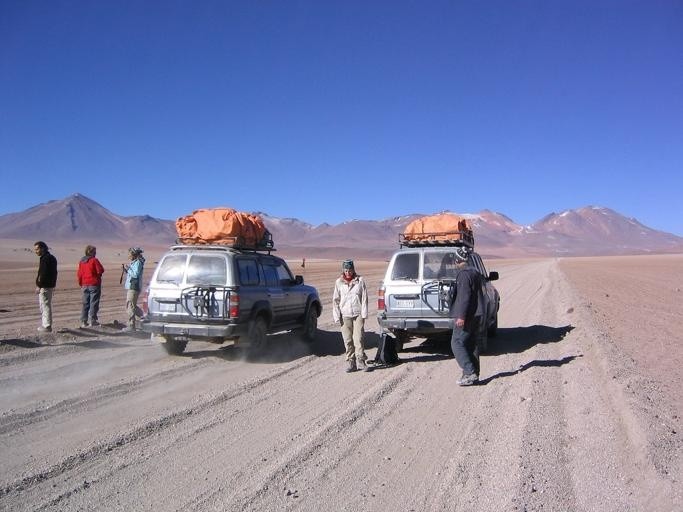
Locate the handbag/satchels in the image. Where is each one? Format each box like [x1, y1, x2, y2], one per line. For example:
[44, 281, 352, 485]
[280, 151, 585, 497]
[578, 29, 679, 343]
[374, 329, 400, 368]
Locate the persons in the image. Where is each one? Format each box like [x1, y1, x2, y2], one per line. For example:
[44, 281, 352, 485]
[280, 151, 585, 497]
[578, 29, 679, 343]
[76, 244, 103, 329]
[449, 246, 480, 386]
[331, 258, 370, 373]
[33, 241, 56, 333]
[119, 246, 145, 333]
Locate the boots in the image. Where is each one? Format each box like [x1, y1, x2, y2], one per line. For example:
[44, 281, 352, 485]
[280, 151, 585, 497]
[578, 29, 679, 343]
[356, 358, 370, 371]
[345, 358, 357, 372]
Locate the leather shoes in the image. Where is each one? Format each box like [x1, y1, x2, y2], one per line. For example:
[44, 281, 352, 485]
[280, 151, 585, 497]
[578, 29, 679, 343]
[89, 319, 99, 326]
[80, 320, 89, 328]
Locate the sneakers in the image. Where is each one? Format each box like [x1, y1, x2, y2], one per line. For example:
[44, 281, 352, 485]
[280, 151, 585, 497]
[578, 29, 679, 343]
[123, 327, 137, 333]
[455, 373, 480, 386]
[37, 325, 52, 332]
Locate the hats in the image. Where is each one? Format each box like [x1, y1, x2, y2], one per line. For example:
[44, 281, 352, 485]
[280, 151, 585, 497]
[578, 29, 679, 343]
[128, 246, 144, 255]
[342, 259, 354, 270]
[454, 245, 469, 262]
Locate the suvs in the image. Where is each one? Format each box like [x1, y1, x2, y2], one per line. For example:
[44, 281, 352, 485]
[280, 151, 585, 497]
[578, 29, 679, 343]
[137, 239, 325, 356]
[377, 233, 502, 354]
